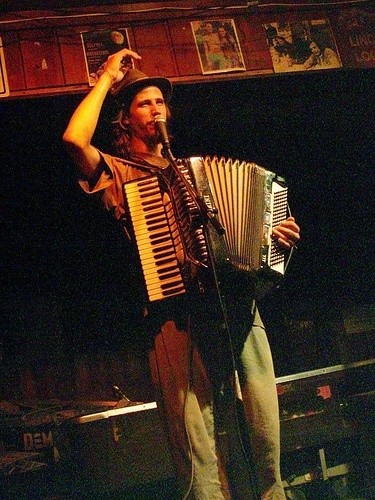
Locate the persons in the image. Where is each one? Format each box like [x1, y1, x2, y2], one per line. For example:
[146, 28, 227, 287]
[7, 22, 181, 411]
[202, 23, 243, 70]
[264, 20, 340, 69]
[61, 49, 301, 500]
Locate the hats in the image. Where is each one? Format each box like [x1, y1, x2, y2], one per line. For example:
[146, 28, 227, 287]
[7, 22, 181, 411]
[94, 68, 172, 137]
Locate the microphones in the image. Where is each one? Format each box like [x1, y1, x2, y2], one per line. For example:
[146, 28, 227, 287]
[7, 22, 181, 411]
[154, 114, 171, 149]
